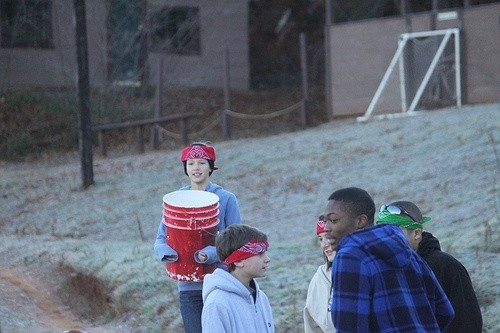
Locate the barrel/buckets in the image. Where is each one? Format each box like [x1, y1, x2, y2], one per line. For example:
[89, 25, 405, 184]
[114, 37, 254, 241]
[161, 190, 220, 283]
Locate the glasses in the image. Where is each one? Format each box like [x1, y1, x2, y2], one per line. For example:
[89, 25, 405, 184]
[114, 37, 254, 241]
[379, 204, 418, 223]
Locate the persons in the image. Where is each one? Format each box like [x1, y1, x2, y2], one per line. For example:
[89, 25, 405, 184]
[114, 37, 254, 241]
[154, 142, 241, 333]
[201, 224, 276, 333]
[323, 187, 455, 333]
[376, 201, 483, 333]
[304, 215, 336, 333]
